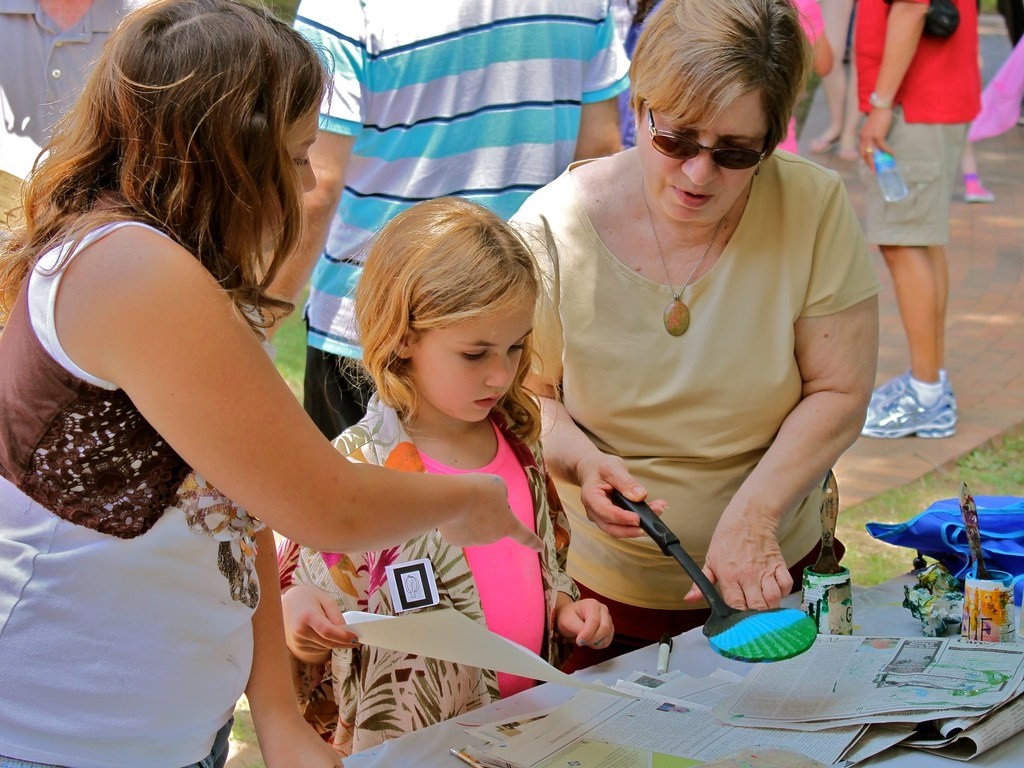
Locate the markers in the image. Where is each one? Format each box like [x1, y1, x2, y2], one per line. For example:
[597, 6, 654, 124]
[655, 632, 673, 675]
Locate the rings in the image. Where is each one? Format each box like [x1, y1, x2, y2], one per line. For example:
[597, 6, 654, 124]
[866, 148, 874, 153]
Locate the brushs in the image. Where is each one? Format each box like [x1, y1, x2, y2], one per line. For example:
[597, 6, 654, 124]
[812, 467, 844, 573]
[960, 482, 990, 578]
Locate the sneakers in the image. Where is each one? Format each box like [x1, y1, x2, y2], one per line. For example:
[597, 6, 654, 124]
[856, 366, 958, 438]
[964, 178, 996, 203]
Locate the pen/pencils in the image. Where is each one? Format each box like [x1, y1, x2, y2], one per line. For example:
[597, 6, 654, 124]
[449, 746, 486, 768]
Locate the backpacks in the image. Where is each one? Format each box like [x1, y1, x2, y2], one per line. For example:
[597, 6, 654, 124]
[865, 495, 1024, 608]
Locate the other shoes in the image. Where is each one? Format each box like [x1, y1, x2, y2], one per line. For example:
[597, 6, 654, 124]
[837, 142, 859, 160]
[809, 134, 843, 154]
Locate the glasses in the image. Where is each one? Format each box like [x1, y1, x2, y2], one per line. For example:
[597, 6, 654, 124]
[642, 99, 766, 170]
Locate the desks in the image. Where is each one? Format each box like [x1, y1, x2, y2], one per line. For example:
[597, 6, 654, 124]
[339, 563, 1023, 768]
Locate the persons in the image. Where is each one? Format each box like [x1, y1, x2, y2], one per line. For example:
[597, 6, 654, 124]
[0, 1, 546, 768]
[778, 0, 835, 154]
[812, 0, 864, 160]
[252, 3, 632, 442]
[852, 0, 983, 437]
[504, 1, 881, 675]
[1, 1, 127, 148]
[275, 196, 615, 761]
[960, 141, 997, 204]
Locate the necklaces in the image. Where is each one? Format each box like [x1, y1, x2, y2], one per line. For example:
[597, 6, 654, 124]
[642, 179, 723, 336]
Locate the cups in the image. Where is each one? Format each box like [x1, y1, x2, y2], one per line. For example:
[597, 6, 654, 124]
[1017, 589, 1024, 638]
[801, 565, 853, 636]
[962, 570, 1016, 643]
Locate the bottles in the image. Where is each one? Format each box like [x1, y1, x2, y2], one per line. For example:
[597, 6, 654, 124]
[871, 147, 908, 203]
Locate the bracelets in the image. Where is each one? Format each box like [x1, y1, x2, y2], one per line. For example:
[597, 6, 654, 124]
[869, 93, 893, 110]
